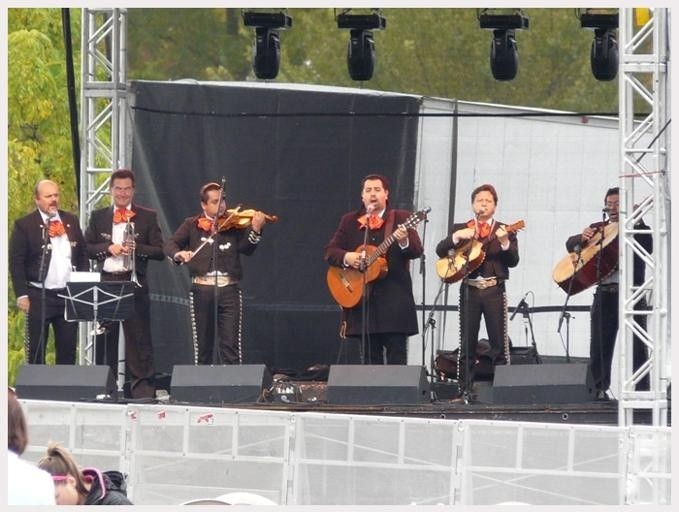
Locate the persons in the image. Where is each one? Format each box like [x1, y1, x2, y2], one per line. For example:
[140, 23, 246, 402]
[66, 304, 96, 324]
[324, 174, 424, 365]
[436, 184, 519, 365]
[566, 187, 653, 400]
[12, 180, 90, 365]
[8, 387, 134, 505]
[85, 169, 164, 397]
[162, 183, 267, 364]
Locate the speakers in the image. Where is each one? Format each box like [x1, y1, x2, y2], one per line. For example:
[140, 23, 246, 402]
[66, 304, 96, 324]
[492, 363, 597, 405]
[14, 364, 118, 404]
[171, 364, 273, 404]
[327, 364, 431, 405]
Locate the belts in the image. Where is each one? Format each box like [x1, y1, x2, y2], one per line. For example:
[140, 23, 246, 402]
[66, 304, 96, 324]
[463, 278, 497, 290]
[191, 274, 240, 287]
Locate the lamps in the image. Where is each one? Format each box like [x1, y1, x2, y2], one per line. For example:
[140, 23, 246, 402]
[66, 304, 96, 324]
[239, 8, 386, 82]
[475, 8, 620, 82]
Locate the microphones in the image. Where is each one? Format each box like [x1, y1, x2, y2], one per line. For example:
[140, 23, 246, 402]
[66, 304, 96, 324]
[423, 205, 432, 213]
[510, 293, 528, 321]
[602, 205, 610, 213]
[367, 204, 375, 213]
[475, 207, 485, 215]
[574, 245, 582, 253]
[46, 213, 56, 222]
[221, 176, 227, 198]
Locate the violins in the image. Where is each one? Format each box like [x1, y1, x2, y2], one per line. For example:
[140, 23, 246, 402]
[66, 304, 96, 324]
[216, 208, 278, 232]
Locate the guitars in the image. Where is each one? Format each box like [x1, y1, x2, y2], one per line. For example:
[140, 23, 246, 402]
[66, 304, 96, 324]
[436, 221, 524, 284]
[554, 205, 643, 295]
[327, 206, 431, 309]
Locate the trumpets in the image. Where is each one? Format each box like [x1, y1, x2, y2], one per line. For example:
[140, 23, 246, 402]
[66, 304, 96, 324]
[122, 215, 143, 289]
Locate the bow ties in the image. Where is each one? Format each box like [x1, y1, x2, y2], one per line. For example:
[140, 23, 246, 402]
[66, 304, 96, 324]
[112, 208, 136, 224]
[48, 220, 66, 238]
[197, 218, 212, 232]
[357, 213, 385, 230]
[466, 220, 491, 238]
